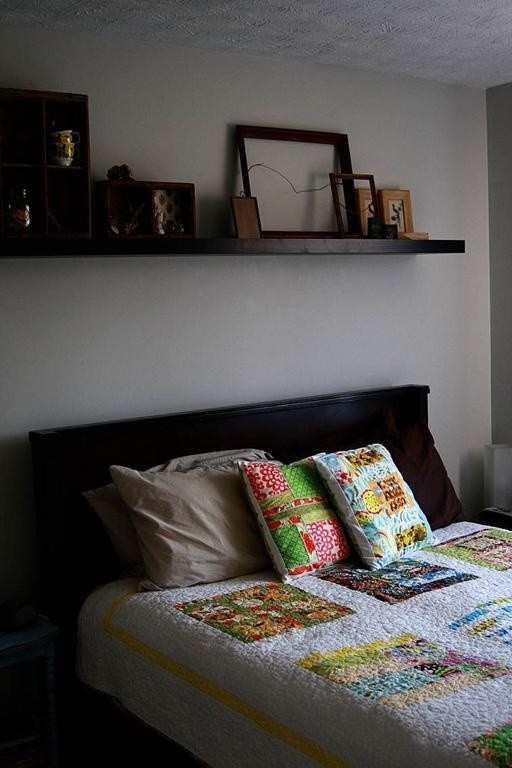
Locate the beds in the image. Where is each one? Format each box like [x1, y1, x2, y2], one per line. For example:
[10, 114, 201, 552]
[28, 383, 512, 768]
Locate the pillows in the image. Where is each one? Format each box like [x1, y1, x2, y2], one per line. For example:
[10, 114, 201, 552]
[237, 453, 354, 584]
[110, 459, 284, 592]
[83, 449, 272, 579]
[311, 444, 442, 572]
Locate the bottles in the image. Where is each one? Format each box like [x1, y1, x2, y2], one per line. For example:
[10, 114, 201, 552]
[9, 187, 32, 235]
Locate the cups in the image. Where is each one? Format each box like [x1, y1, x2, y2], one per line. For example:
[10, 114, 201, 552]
[49, 128, 81, 167]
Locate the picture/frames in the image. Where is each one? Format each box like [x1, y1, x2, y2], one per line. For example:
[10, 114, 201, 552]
[357, 188, 414, 240]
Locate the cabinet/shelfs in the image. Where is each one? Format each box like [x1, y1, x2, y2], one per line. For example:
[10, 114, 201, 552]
[0, 86, 94, 239]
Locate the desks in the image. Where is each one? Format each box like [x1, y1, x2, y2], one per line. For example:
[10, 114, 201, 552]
[0, 620, 62, 768]
